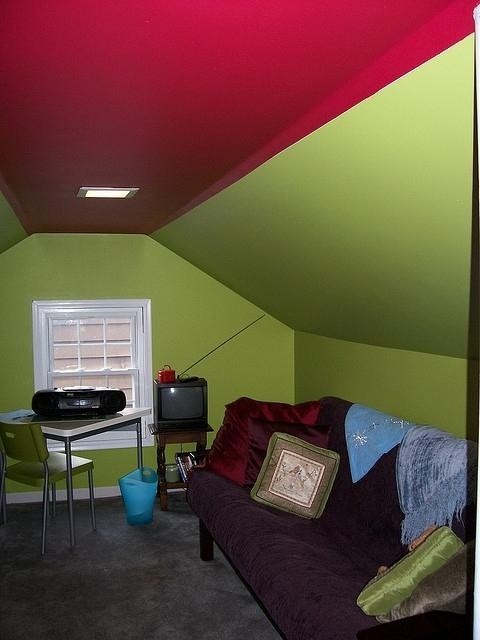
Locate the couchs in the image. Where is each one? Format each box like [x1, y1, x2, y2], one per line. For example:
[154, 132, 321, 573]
[187, 391, 473, 638]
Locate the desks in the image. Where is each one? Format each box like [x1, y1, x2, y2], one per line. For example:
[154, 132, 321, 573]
[148, 422, 213, 509]
[0, 408, 151, 546]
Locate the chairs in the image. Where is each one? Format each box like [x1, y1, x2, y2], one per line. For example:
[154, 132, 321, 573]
[0, 420, 98, 555]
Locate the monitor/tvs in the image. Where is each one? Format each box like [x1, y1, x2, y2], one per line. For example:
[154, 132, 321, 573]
[153, 380, 208, 430]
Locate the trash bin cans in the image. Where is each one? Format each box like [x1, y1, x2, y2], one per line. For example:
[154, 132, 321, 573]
[119, 468, 158, 526]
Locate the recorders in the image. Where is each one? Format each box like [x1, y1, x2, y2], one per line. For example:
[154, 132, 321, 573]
[32, 385, 126, 416]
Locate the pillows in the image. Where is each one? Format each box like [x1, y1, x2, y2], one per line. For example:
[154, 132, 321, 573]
[353, 525, 467, 624]
[248, 431, 343, 521]
